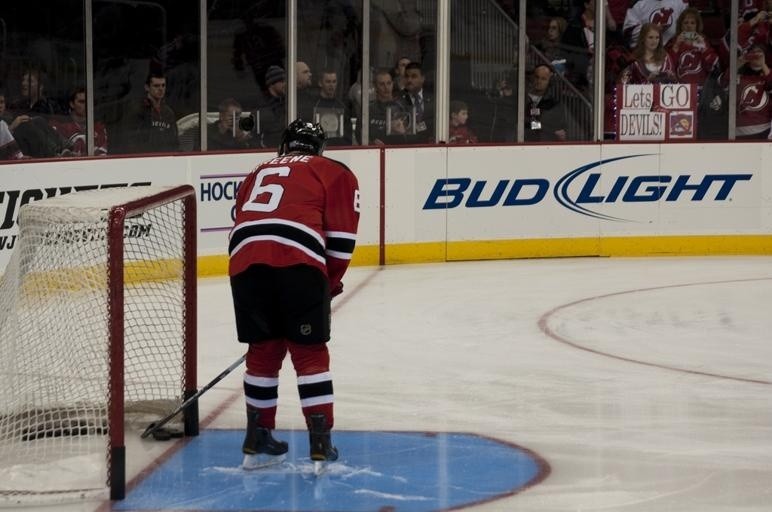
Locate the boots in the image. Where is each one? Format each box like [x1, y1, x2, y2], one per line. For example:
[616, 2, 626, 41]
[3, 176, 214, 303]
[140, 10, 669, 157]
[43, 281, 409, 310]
[309, 414, 339, 462]
[242, 411, 289, 455]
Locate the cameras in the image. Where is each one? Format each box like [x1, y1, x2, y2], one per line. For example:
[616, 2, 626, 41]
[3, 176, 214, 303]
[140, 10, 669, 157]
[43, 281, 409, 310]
[681, 32, 696, 41]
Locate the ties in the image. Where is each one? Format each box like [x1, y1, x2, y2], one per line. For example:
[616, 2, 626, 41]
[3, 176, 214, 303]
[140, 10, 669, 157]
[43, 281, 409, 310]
[412, 94, 424, 122]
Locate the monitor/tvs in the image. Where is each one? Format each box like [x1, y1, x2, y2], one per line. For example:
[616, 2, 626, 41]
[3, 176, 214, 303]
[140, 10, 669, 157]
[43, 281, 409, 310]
[234, 111, 257, 135]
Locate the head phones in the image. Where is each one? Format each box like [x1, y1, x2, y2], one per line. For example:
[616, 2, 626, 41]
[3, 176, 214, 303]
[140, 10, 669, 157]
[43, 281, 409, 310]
[529, 63, 556, 88]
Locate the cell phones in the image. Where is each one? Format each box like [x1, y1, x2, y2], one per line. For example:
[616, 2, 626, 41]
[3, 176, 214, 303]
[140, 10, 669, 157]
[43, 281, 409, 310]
[745, 52, 760, 60]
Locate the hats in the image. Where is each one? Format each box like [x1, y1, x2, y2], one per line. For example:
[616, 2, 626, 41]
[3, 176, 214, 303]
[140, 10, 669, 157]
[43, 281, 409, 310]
[264, 65, 286, 85]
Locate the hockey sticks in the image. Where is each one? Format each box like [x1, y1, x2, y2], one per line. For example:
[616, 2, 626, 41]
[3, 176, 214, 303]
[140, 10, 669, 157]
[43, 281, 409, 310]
[142, 353, 247, 438]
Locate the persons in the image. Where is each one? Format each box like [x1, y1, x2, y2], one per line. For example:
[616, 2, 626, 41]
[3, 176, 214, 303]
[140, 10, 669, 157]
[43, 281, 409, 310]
[227, 118, 359, 461]
[1, 1, 770, 158]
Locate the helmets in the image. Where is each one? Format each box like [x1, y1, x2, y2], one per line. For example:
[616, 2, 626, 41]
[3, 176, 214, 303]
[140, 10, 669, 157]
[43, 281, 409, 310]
[278, 118, 328, 158]
